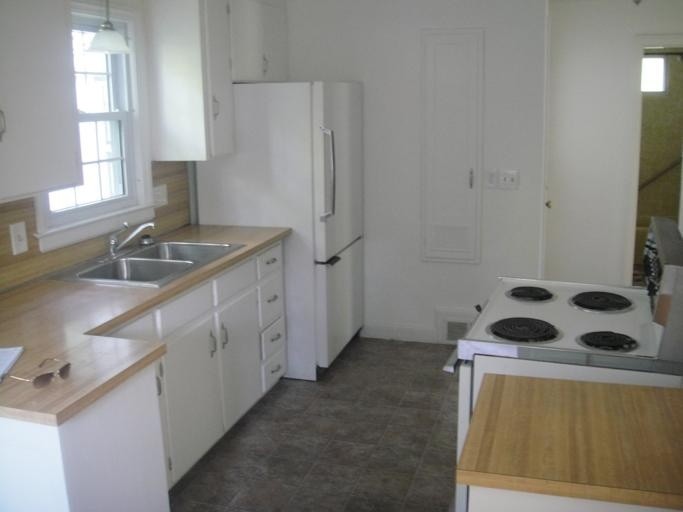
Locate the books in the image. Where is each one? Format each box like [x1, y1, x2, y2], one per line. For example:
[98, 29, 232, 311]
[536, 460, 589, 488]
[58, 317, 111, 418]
[0, 346, 25, 380]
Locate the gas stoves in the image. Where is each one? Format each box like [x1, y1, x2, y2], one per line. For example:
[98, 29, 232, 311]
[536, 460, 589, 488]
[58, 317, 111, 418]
[466, 275, 657, 359]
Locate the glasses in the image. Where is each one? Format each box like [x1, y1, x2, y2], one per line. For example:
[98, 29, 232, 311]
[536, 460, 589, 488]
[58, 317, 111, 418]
[10, 357, 71, 388]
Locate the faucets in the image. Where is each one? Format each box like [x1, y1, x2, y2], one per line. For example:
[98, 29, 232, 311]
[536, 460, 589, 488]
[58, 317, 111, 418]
[107, 221, 159, 258]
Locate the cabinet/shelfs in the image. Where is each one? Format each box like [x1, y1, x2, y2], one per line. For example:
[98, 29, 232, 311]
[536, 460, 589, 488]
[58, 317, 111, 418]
[229, 0, 289, 83]
[143, 0, 235, 163]
[0, 0, 83, 204]
[420, 25, 486, 266]
[0, 239, 289, 511]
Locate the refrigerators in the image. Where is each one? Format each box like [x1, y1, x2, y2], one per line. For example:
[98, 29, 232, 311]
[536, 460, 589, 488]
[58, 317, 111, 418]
[196, 83, 367, 382]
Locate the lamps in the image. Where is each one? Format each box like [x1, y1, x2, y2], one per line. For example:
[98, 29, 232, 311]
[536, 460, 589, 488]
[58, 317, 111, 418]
[86, 0, 132, 54]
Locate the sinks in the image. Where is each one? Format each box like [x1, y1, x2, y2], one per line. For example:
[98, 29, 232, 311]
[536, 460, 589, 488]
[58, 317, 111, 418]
[121, 240, 233, 264]
[77, 257, 189, 286]
